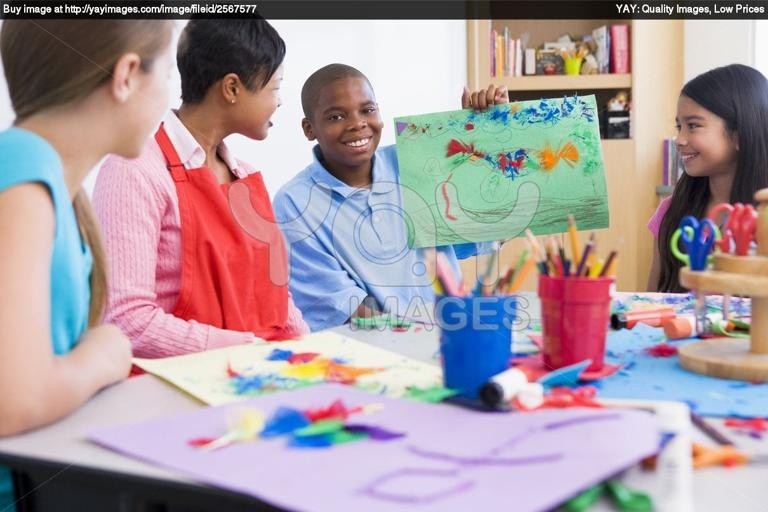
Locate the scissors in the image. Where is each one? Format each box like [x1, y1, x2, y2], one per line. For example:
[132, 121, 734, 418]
[669, 200, 758, 336]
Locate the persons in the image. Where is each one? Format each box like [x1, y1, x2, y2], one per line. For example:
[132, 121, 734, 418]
[273, 58, 512, 334]
[645, 63, 767, 294]
[0, 14, 184, 511]
[91, 17, 312, 381]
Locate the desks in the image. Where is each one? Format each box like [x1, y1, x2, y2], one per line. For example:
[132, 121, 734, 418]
[1, 294, 767, 512]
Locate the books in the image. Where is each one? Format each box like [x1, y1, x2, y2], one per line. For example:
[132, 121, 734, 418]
[489, 22, 630, 77]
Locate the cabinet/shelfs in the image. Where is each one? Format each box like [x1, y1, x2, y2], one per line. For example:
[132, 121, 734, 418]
[467, 20, 685, 292]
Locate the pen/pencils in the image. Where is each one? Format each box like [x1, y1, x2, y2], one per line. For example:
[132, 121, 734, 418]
[426, 213, 619, 296]
[691, 410, 732, 445]
